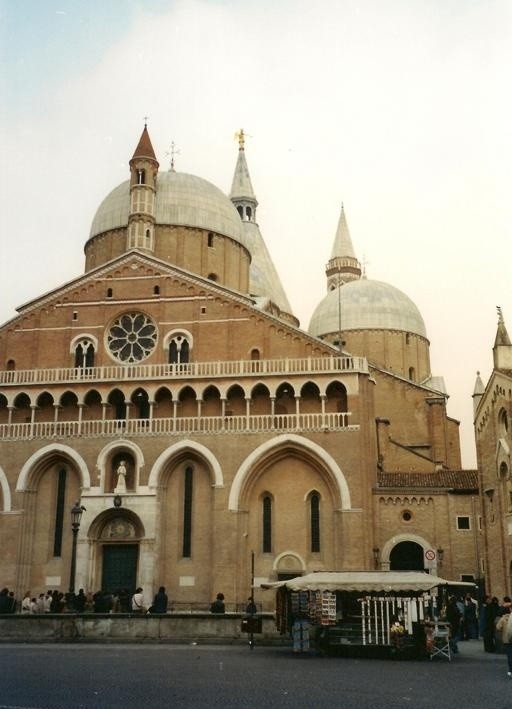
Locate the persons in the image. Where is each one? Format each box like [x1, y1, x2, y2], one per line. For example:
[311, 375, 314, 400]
[441, 591, 511, 677]
[114, 460, 128, 493]
[1, 586, 132, 615]
[131, 587, 146, 613]
[246, 596, 257, 613]
[147, 586, 169, 614]
[208, 592, 224, 615]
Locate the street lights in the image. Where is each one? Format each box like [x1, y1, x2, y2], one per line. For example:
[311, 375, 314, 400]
[67, 500, 87, 613]
